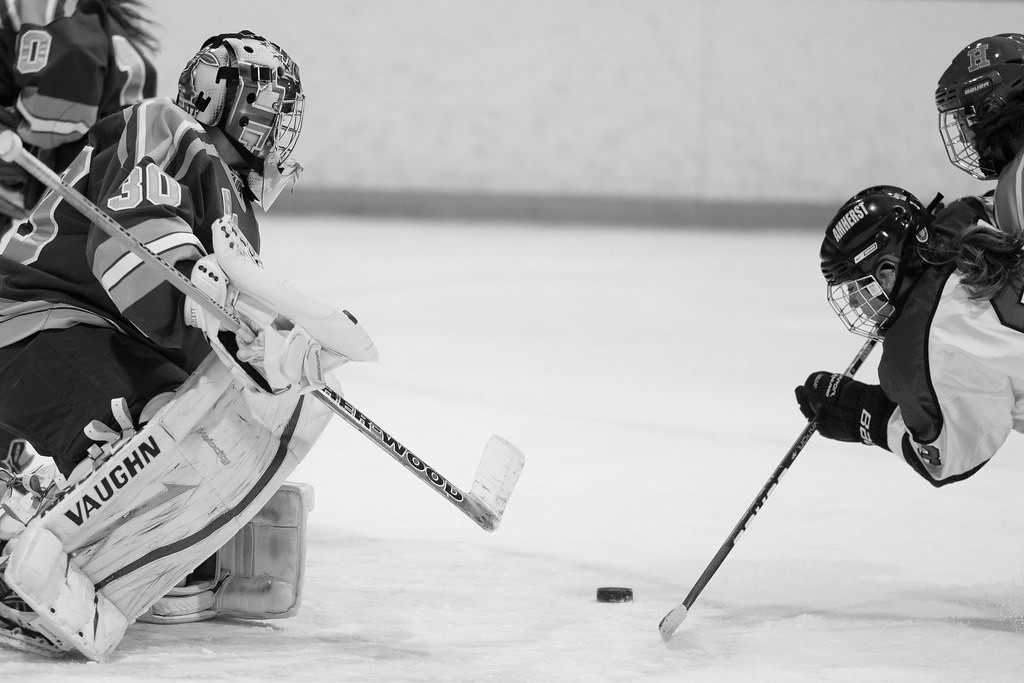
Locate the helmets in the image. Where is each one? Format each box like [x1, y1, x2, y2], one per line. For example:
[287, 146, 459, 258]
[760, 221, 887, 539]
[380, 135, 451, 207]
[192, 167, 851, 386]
[935, 33, 1024, 180]
[172, 30, 305, 213]
[819, 186, 936, 342]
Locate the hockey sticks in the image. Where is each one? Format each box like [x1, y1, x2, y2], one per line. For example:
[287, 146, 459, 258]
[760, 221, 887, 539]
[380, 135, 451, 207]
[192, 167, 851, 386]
[0, 124, 529, 536]
[657, 320, 890, 646]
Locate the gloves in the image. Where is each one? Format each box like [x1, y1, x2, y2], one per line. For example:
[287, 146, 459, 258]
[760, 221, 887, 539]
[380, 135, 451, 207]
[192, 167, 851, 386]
[795, 371, 898, 453]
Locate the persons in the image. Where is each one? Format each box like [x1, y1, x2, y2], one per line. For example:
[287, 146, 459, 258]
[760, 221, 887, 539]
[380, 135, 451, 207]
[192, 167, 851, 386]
[796, 31, 1024, 487]
[1, 0, 381, 661]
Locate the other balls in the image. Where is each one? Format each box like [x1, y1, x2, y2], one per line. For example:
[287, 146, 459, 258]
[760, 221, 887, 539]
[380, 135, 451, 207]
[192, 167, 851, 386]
[597, 587, 634, 604]
